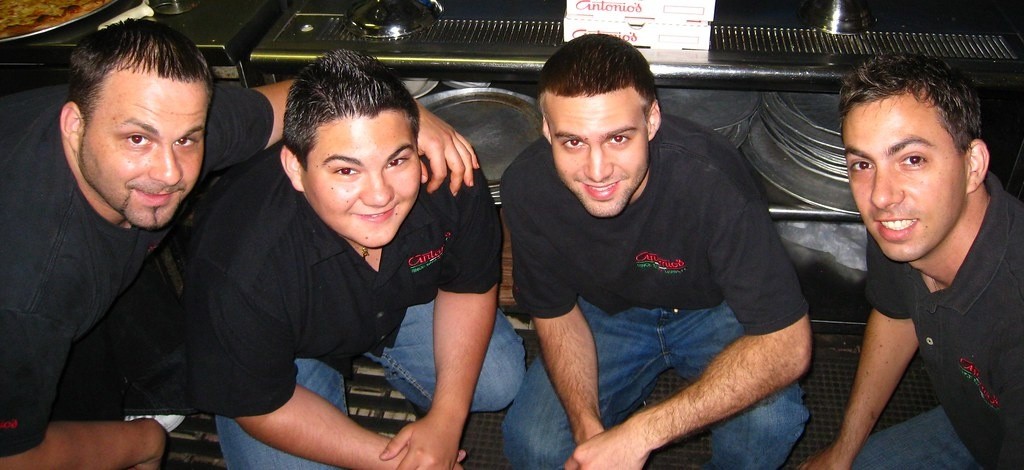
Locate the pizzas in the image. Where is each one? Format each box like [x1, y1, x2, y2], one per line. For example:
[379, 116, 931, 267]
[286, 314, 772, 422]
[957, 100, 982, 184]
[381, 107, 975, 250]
[0, 0, 110, 37]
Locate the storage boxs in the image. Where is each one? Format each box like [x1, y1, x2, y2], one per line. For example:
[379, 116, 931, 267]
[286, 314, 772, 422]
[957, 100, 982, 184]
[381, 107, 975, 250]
[564, 0, 716, 50]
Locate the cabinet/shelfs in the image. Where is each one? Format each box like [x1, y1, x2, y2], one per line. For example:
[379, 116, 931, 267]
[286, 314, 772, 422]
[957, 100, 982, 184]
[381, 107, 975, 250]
[250, 0, 1024, 335]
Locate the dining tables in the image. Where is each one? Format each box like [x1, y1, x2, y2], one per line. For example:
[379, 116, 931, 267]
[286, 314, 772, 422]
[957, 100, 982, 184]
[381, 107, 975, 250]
[0, 0, 282, 97]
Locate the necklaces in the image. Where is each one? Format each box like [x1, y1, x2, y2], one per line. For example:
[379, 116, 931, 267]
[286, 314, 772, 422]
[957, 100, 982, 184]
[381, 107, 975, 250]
[362, 246, 370, 260]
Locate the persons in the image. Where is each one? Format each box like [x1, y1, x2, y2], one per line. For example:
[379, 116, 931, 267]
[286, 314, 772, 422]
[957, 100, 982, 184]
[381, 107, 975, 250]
[184, 49, 526, 470]
[499, 33, 812, 470]
[798, 53, 1024, 470]
[0, 19, 479, 470]
